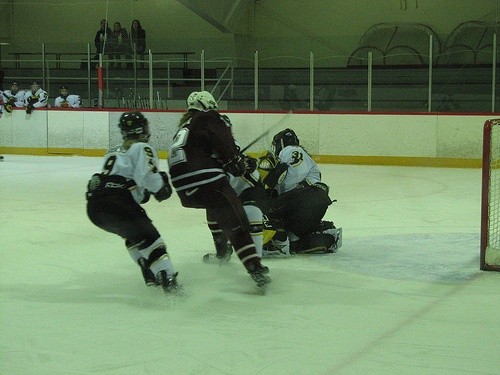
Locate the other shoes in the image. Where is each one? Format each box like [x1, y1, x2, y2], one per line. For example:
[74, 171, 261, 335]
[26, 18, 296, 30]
[137, 258, 158, 287]
[156, 269, 184, 297]
[263, 237, 290, 259]
[322, 220, 343, 254]
[203, 250, 234, 266]
[248, 263, 272, 289]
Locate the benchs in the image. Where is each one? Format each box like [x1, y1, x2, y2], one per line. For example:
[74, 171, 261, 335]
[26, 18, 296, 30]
[8, 52, 197, 70]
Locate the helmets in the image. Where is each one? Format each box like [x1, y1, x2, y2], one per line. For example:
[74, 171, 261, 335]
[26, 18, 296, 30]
[117, 111, 150, 139]
[271, 128, 300, 157]
[187, 91, 218, 112]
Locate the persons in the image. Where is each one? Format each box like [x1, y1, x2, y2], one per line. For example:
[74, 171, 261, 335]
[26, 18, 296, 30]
[228, 128, 343, 257]
[85, 112, 192, 311]
[55, 85, 81, 108]
[23, 81, 51, 114]
[89, 19, 146, 73]
[168, 91, 272, 295]
[1, 81, 26, 113]
